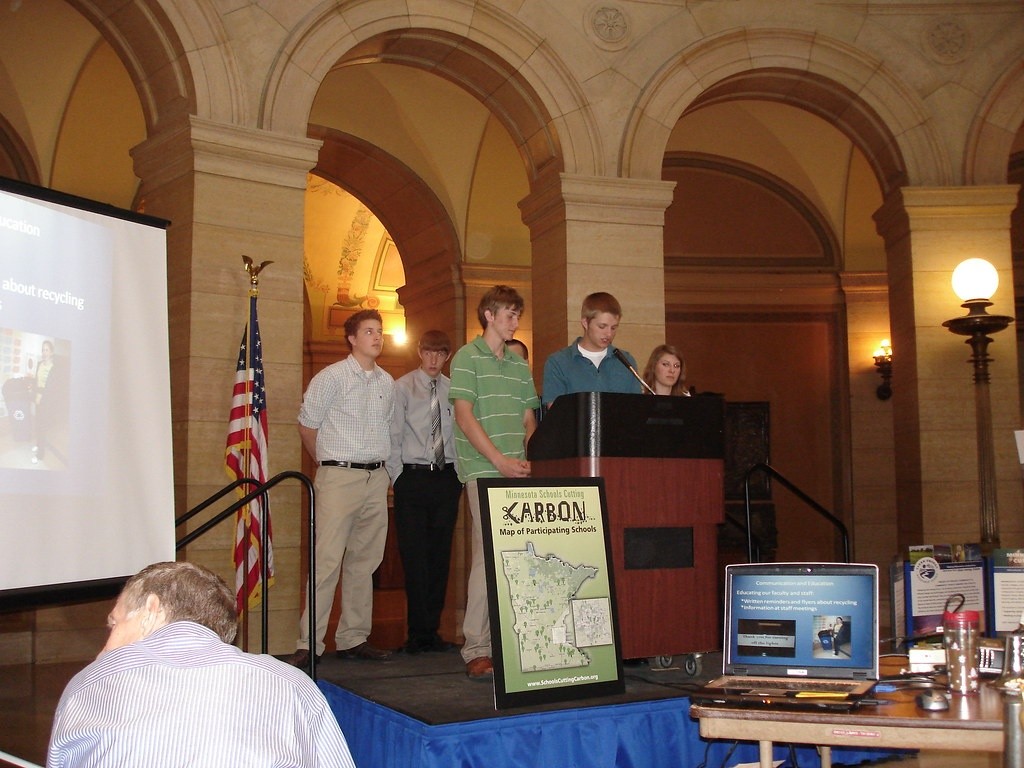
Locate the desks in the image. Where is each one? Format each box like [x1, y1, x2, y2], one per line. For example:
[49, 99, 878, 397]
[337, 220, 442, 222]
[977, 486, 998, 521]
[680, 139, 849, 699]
[691, 652, 1023, 768]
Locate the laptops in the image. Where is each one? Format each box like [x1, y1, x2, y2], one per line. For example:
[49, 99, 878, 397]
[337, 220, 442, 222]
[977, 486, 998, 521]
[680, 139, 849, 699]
[689, 562, 879, 713]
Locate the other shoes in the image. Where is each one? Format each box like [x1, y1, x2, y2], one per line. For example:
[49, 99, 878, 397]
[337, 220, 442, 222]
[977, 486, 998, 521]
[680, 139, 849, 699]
[397, 640, 426, 656]
[426, 635, 456, 653]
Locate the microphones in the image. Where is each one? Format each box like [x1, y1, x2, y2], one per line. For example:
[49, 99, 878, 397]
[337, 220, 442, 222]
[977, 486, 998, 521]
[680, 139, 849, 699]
[613, 348, 656, 395]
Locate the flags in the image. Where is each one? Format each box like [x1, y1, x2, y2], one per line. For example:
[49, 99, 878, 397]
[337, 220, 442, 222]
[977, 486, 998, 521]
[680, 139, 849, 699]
[225, 288, 275, 626]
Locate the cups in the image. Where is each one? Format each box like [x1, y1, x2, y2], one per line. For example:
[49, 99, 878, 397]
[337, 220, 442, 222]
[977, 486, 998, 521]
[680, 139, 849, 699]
[943, 611, 979, 693]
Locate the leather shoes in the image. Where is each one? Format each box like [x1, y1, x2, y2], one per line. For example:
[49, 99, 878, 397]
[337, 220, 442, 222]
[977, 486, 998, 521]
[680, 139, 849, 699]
[286, 649, 321, 669]
[336, 643, 393, 660]
[466, 657, 494, 681]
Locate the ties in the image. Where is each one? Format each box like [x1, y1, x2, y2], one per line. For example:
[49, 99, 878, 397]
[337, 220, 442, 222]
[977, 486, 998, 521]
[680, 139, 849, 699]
[429, 379, 445, 470]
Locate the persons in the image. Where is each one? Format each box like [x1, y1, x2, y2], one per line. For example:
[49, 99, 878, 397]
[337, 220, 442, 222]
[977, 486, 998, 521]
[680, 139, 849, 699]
[384, 330, 460, 653]
[46, 562, 356, 768]
[28, 341, 62, 464]
[831, 617, 846, 656]
[641, 344, 690, 396]
[505, 339, 528, 365]
[541, 293, 641, 409]
[287, 309, 396, 668]
[447, 283, 541, 679]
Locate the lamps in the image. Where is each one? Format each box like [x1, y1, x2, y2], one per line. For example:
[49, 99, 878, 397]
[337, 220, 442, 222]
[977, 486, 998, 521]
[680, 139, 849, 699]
[872, 338, 893, 399]
[942, 259, 1015, 540]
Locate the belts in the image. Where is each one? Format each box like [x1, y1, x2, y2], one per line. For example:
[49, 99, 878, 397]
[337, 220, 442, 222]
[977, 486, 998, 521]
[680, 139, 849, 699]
[322, 460, 385, 470]
[405, 463, 454, 471]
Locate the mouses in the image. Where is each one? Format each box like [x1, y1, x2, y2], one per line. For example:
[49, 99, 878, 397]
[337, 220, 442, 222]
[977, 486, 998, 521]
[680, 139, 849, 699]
[915, 689, 949, 711]
